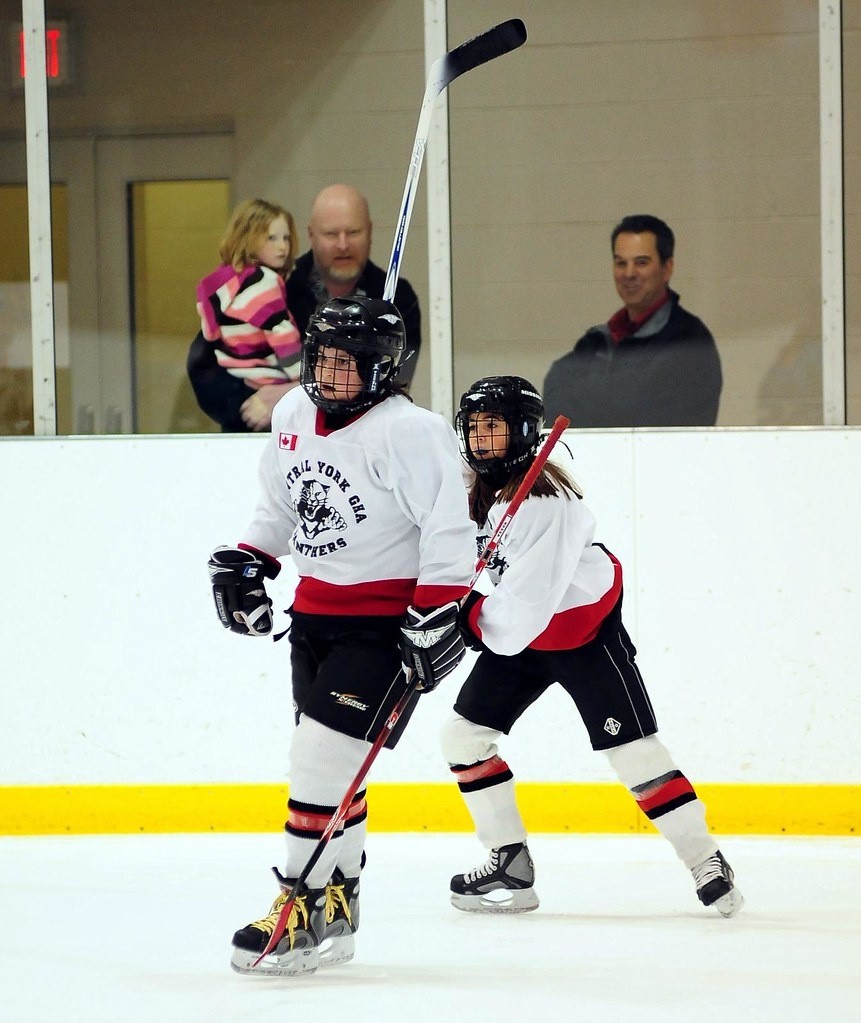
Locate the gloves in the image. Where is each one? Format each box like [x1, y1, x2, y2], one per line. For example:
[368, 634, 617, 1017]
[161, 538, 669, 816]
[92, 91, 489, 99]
[396, 601, 467, 693]
[457, 587, 489, 652]
[207, 544, 282, 636]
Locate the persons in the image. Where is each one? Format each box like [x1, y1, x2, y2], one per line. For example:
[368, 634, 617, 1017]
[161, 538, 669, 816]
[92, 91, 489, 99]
[441, 377, 745, 919]
[196, 199, 306, 389]
[542, 214, 722, 429]
[186, 183, 422, 435]
[206, 296, 475, 977]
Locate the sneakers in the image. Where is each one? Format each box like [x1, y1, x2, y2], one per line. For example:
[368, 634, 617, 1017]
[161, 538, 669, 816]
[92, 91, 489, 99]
[690, 849, 744, 918]
[230, 867, 326, 977]
[317, 850, 366, 969]
[450, 840, 539, 912]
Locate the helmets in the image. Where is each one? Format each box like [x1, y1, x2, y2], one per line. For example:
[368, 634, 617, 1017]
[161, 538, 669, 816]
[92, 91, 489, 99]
[455, 375, 545, 475]
[299, 295, 407, 415]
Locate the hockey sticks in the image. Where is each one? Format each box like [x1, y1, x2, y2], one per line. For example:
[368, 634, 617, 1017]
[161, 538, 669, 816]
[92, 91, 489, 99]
[382, 17, 528, 301]
[251, 414, 570, 969]
